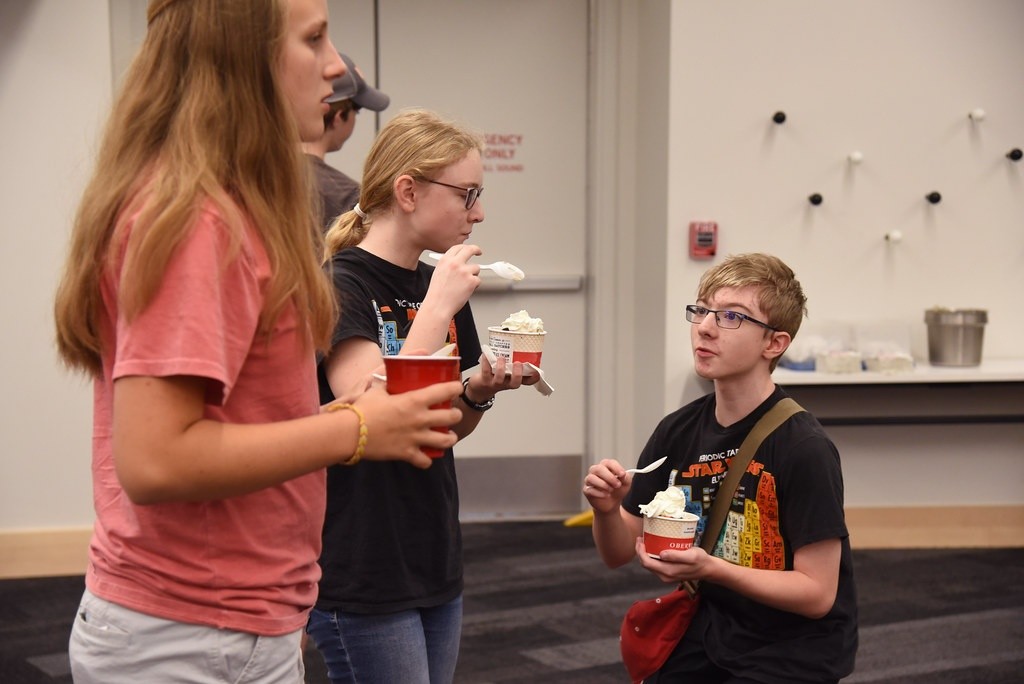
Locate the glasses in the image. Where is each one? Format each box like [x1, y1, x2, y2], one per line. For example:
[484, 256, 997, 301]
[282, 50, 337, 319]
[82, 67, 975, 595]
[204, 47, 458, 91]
[413, 175, 484, 211]
[685, 304, 782, 335]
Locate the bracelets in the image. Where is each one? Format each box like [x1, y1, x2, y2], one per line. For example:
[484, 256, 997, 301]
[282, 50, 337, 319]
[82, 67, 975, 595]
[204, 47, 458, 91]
[461, 377, 495, 412]
[328, 404, 367, 465]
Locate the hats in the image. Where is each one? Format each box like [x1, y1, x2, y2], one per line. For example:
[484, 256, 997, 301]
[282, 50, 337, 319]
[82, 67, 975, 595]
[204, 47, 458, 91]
[320, 52, 389, 111]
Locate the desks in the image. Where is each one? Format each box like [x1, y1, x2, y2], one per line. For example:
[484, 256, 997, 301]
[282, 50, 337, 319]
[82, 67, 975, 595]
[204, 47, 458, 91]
[769, 359, 1024, 425]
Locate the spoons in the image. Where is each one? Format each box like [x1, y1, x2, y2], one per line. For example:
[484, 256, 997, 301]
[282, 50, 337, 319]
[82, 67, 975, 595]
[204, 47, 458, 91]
[586, 456, 667, 490]
[429, 253, 524, 281]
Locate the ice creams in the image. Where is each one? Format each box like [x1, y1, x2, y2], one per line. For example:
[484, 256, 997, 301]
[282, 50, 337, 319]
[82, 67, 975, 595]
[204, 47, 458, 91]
[637, 486, 686, 519]
[501, 309, 544, 334]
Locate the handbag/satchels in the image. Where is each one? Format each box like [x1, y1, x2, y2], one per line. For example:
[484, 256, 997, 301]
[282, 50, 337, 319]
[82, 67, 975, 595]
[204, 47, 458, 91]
[619, 580, 699, 683]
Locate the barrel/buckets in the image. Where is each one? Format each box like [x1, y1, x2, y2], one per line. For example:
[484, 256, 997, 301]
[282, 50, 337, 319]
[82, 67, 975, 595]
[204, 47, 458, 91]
[924, 309, 987, 367]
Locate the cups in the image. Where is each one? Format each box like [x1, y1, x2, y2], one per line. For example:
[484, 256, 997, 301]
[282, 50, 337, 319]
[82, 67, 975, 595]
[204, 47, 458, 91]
[643, 512, 700, 559]
[383, 356, 461, 457]
[488, 327, 547, 376]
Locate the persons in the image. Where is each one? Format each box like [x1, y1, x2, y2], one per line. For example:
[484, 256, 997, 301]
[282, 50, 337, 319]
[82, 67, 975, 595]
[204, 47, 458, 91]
[583, 253, 859, 684]
[307, 110, 542, 684]
[50, 0, 466, 684]
[301, 53, 390, 250]
